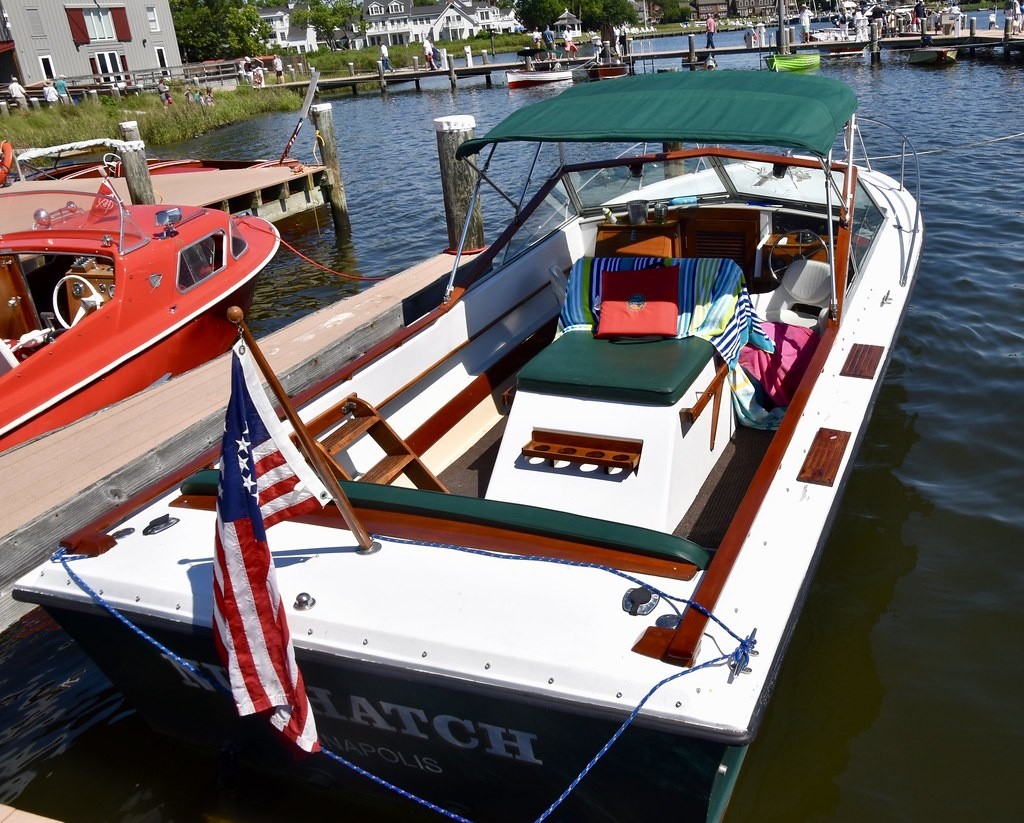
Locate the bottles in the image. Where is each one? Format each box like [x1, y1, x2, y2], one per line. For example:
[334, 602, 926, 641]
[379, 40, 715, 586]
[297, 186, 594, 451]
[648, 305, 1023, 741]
[603, 207, 616, 223]
[669, 197, 697, 205]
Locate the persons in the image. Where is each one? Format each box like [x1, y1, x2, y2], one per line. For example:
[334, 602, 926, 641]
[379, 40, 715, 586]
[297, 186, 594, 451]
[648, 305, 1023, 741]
[8, 77, 31, 112]
[600, 21, 628, 57]
[704, 13, 716, 49]
[272, 53, 286, 84]
[380, 42, 396, 74]
[244, 56, 254, 87]
[183, 87, 217, 107]
[798, 4, 814, 43]
[158, 78, 173, 114]
[1012, 0, 1024, 35]
[43, 75, 75, 106]
[422, 38, 440, 70]
[833, 0, 931, 43]
[532, 24, 578, 62]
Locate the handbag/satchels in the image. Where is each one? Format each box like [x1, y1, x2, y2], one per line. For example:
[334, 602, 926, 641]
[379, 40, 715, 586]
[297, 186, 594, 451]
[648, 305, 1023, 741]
[1013, 19, 1018, 27]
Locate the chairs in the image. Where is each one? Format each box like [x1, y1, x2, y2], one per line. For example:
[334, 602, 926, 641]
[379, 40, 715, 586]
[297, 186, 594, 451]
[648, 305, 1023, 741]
[750, 259, 831, 334]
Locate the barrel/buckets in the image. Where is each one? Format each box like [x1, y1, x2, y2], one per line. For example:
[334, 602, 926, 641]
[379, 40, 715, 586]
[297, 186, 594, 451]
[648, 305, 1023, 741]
[626, 200, 649, 224]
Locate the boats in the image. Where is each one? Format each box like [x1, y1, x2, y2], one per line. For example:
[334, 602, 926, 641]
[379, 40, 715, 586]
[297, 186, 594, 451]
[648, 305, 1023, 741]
[0, 139, 284, 456]
[9, 68, 925, 823]
[906, 33, 958, 65]
[505, 48, 573, 88]
[763, 52, 821, 73]
[584, 60, 630, 81]
[801, 5, 964, 55]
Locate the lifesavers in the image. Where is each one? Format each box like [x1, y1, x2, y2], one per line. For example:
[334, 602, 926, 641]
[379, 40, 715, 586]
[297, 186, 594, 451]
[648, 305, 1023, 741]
[0, 140, 13, 186]
[744, 32, 758, 46]
[253, 72, 263, 85]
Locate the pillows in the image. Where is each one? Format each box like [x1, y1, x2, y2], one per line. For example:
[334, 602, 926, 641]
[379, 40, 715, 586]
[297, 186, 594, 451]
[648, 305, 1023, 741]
[597, 261, 682, 337]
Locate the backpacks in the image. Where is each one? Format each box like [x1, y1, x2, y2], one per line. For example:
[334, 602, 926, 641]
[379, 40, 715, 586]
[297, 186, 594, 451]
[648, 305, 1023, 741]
[840, 13, 846, 24]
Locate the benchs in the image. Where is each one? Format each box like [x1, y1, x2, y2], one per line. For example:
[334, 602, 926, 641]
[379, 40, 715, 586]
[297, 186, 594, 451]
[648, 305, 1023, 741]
[539, 244, 747, 360]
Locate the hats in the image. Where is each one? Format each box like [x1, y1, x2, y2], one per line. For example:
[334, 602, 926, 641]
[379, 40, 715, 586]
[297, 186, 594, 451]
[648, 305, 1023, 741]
[800, 4, 807, 9]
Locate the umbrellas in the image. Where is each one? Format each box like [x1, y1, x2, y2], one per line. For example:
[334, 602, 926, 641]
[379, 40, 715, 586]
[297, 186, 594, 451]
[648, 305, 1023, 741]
[838, 1, 857, 16]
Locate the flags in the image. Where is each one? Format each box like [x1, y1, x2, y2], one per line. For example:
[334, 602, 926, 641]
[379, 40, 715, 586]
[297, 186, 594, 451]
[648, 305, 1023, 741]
[212, 341, 334, 754]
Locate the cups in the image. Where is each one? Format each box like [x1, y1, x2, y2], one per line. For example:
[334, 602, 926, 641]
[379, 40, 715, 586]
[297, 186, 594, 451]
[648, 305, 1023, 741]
[655, 204, 668, 224]
